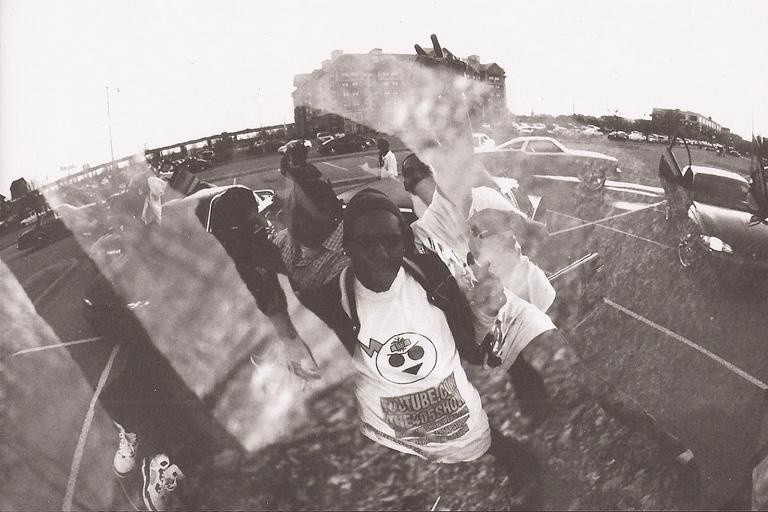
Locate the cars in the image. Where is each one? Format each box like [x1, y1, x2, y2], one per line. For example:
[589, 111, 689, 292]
[506, 119, 602, 145]
[352, 134, 375, 148]
[1, 146, 218, 251]
[316, 137, 371, 154]
[655, 135, 767, 288]
[83, 182, 283, 333]
[81, 179, 215, 269]
[472, 131, 495, 155]
[606, 128, 743, 158]
[474, 136, 623, 182]
[233, 136, 286, 156]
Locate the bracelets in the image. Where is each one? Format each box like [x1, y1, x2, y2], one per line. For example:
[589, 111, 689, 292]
[670, 447, 691, 473]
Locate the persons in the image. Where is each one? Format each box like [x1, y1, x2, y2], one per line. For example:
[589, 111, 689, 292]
[130, 32, 700, 511]
[110, 418, 182, 511]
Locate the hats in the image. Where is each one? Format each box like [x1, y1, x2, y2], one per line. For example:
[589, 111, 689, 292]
[342, 187, 409, 239]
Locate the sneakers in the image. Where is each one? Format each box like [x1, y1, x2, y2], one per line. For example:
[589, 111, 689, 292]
[112, 430, 141, 479]
[141, 450, 186, 512]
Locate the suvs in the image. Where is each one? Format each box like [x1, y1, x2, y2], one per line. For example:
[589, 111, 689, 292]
[277, 139, 312, 156]
[316, 131, 346, 142]
